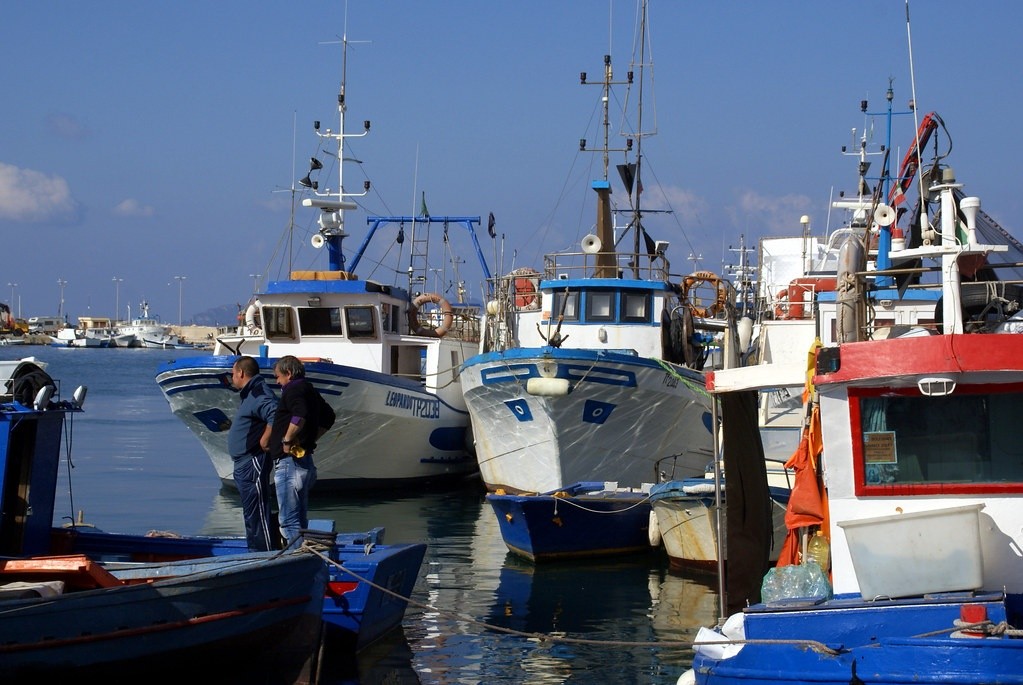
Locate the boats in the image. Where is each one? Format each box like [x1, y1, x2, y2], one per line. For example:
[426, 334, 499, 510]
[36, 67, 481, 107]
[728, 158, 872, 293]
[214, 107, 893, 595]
[680, 169, 1023, 685]
[155, 0, 488, 500]
[704, 92, 1023, 507]
[0, 525, 330, 685]
[458, 0, 722, 561]
[0, 353, 427, 639]
[39, 320, 179, 348]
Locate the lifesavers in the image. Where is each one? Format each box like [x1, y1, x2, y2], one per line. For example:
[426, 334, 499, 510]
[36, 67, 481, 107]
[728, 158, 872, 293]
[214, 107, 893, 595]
[775, 288, 790, 320]
[680, 270, 728, 318]
[407, 292, 454, 339]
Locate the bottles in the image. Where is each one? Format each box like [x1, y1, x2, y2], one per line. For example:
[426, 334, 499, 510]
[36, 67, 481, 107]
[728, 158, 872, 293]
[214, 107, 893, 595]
[807, 531, 829, 573]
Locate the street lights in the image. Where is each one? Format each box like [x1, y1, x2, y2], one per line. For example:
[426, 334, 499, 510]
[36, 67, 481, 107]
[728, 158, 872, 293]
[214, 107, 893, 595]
[5, 281, 17, 317]
[687, 256, 705, 273]
[57, 279, 68, 326]
[175, 275, 186, 325]
[112, 276, 124, 327]
[430, 266, 443, 309]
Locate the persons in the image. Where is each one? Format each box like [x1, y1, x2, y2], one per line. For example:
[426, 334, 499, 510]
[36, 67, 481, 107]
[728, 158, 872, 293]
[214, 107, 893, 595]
[228, 356, 280, 554]
[267, 356, 336, 552]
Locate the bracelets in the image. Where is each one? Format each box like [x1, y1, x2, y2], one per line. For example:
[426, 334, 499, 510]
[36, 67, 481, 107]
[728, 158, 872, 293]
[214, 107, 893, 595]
[282, 438, 292, 445]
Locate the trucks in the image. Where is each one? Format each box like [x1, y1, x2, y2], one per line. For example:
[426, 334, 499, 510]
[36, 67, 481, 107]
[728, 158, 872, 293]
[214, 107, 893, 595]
[0, 318, 28, 336]
[28, 316, 58, 335]
